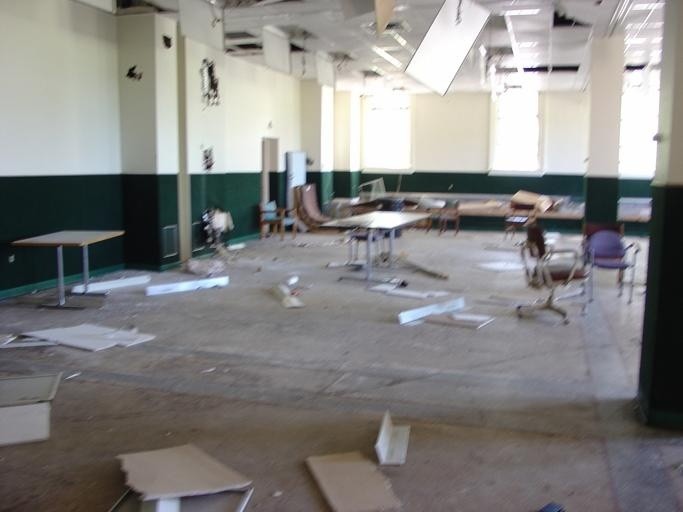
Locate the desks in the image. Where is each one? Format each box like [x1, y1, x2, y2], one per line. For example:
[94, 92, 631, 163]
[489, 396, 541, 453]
[11, 228, 126, 311]
[319, 210, 432, 286]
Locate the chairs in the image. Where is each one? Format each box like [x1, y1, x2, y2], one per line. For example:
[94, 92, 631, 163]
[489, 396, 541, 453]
[259, 188, 541, 240]
[347, 207, 384, 265]
[516, 216, 588, 326]
[582, 220, 641, 303]
